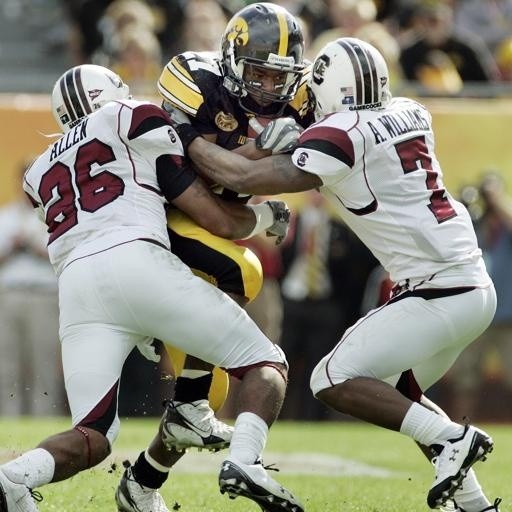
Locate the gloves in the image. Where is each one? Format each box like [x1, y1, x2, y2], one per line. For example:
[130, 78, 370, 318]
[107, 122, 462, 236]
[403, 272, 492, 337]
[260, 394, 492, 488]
[265, 198, 290, 246]
[161, 101, 205, 157]
[135, 336, 164, 363]
[255, 115, 301, 156]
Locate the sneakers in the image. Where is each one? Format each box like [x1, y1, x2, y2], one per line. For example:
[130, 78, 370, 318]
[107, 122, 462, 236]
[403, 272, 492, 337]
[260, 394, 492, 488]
[451, 497, 503, 512]
[114, 467, 172, 512]
[0, 467, 40, 512]
[159, 398, 233, 453]
[427, 416, 494, 509]
[218, 458, 303, 512]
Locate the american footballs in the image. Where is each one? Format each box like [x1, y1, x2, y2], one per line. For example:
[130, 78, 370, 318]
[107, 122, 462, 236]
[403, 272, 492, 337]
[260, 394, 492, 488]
[247, 116, 304, 145]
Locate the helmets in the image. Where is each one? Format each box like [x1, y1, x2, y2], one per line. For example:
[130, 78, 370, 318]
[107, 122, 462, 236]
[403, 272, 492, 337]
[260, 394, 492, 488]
[218, 1, 303, 120]
[307, 37, 391, 120]
[48, 64, 130, 135]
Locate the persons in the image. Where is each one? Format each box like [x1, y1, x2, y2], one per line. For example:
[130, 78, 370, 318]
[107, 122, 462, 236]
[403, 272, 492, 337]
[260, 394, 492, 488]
[163, 38, 503, 512]
[1, 1, 511, 99]
[114, 1, 309, 512]
[0, 62, 307, 512]
